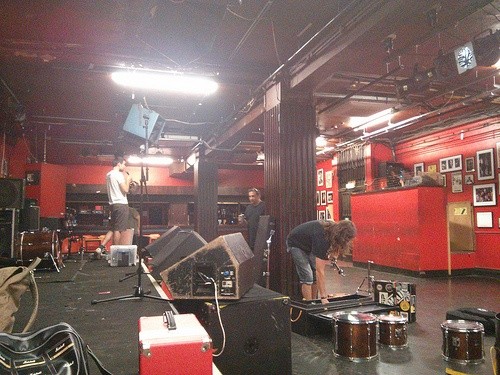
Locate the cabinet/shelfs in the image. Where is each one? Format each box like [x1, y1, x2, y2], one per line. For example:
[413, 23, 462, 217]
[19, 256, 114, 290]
[64, 195, 250, 234]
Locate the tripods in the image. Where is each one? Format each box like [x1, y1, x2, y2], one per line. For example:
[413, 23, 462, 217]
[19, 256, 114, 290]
[90, 151, 173, 306]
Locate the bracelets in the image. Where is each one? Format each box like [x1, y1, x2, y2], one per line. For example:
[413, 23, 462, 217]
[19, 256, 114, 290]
[320, 296, 327, 299]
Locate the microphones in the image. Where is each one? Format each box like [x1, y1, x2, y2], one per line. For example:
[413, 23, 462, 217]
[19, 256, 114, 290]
[126, 170, 130, 175]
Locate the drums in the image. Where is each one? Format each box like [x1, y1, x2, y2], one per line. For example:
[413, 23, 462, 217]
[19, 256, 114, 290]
[19, 230, 62, 270]
[377, 313, 409, 349]
[439, 319, 485, 366]
[332, 313, 378, 363]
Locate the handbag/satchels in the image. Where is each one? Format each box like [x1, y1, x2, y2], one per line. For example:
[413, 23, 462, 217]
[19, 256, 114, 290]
[0, 254, 112, 375]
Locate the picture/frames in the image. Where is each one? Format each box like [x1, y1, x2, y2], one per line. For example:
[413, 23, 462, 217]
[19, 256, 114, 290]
[476, 148, 495, 180]
[413, 162, 425, 177]
[316, 191, 320, 206]
[327, 191, 333, 203]
[326, 207, 333, 221]
[321, 190, 327, 205]
[317, 168, 325, 186]
[438, 154, 463, 174]
[465, 156, 475, 172]
[472, 183, 497, 206]
[318, 210, 326, 221]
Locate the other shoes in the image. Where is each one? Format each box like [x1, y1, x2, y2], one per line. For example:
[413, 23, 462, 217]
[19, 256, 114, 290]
[96, 245, 108, 257]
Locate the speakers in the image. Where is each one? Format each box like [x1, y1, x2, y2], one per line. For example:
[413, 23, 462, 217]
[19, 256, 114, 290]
[0, 177, 40, 231]
[144, 226, 256, 300]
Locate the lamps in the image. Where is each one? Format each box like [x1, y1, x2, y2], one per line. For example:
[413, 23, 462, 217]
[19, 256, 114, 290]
[394, 30, 500, 102]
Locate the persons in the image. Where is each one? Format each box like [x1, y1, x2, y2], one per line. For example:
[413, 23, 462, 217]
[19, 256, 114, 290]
[106, 156, 132, 245]
[286, 219, 356, 304]
[239, 187, 266, 252]
[93, 207, 141, 260]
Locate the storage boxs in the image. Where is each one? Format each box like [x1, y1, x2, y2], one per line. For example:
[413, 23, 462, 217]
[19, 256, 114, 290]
[110, 244, 137, 267]
